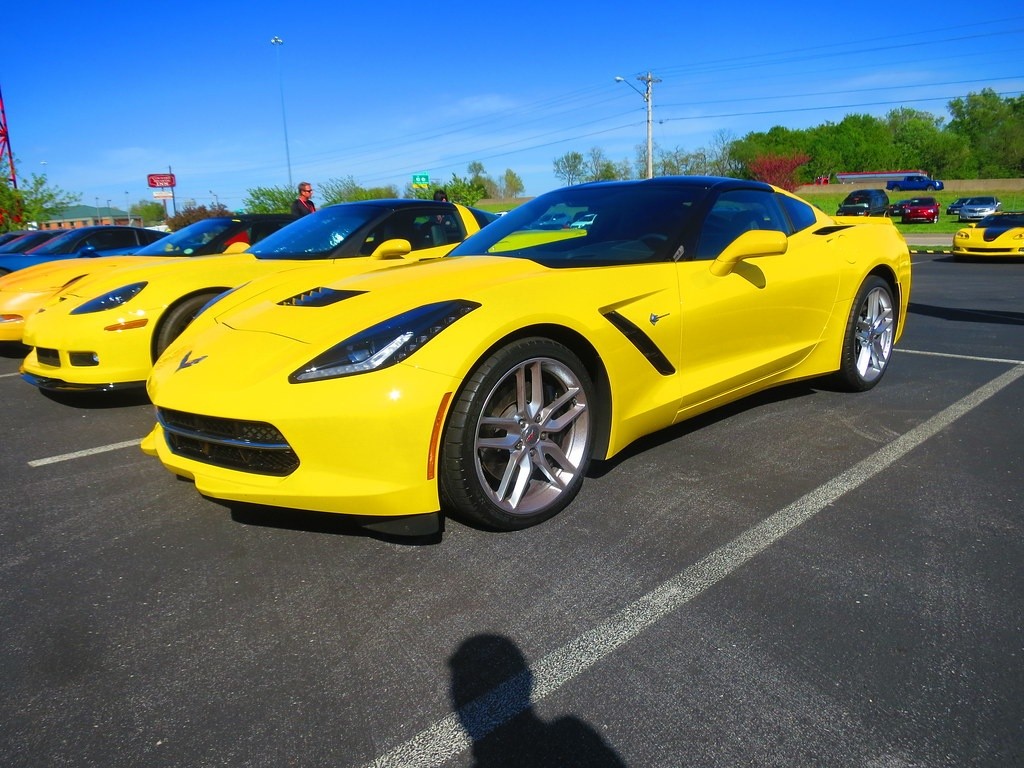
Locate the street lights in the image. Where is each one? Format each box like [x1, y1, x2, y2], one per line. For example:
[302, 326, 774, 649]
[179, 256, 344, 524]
[615, 76, 653, 178]
[271, 36, 294, 191]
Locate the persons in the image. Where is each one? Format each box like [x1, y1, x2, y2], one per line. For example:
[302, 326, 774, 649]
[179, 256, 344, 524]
[291, 182, 316, 217]
[429, 189, 452, 226]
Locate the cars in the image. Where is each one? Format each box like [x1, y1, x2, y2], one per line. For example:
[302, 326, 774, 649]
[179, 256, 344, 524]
[959, 197, 1001, 221]
[889, 200, 910, 216]
[531, 212, 571, 229]
[570, 213, 596, 229]
[947, 197, 972, 214]
[902, 197, 940, 223]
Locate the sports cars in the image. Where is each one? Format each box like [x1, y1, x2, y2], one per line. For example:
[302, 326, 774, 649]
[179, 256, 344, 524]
[0, 200, 588, 394]
[951, 211, 1024, 258]
[139, 174, 912, 534]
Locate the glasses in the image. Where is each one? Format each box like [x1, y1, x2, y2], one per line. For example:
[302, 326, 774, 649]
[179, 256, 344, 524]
[302, 189, 312, 193]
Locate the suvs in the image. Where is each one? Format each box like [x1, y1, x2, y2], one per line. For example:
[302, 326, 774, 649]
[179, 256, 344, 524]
[836, 189, 889, 216]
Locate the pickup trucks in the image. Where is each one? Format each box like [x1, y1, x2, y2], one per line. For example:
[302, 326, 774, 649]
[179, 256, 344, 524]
[885, 175, 944, 192]
